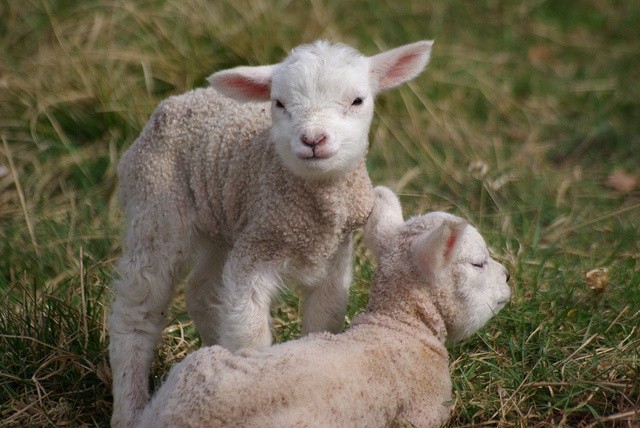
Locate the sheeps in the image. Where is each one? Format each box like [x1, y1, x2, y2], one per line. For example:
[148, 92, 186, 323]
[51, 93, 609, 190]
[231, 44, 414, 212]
[106, 38, 435, 428]
[136, 184, 512, 426]
